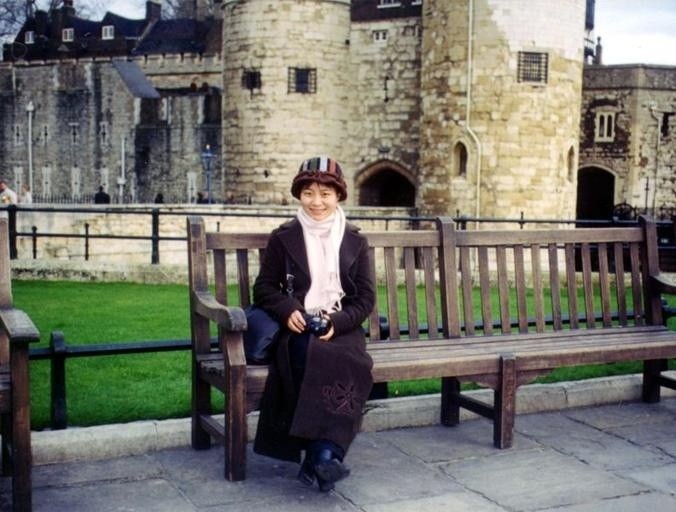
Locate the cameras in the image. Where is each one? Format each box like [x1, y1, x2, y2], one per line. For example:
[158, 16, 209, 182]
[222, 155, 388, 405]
[301, 314, 332, 338]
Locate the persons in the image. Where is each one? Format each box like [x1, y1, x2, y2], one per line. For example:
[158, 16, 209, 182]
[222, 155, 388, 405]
[197, 190, 214, 203]
[224, 190, 235, 205]
[17, 183, 31, 204]
[153, 193, 163, 204]
[252, 157, 377, 492]
[0, 180, 16, 204]
[94, 184, 109, 204]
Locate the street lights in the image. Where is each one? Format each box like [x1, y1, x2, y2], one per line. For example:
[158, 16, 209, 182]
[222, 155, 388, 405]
[199, 143, 218, 204]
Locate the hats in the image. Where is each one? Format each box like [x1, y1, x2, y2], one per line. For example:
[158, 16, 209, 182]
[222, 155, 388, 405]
[290, 155, 349, 201]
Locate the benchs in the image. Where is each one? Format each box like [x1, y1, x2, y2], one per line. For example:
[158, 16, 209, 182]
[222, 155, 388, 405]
[186, 214, 676, 481]
[0, 218, 41, 512]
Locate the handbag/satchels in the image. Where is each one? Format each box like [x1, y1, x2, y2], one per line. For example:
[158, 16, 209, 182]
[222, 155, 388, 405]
[243, 254, 294, 365]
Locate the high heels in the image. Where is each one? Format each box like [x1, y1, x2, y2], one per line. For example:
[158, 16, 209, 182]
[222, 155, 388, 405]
[317, 465, 351, 492]
[297, 450, 344, 487]
